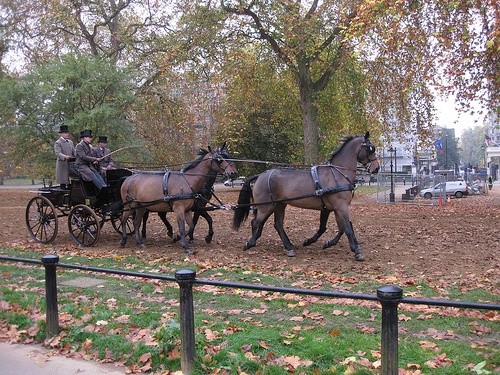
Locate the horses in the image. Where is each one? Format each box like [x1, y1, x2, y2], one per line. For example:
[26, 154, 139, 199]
[231, 129, 380, 261]
[117, 140, 241, 253]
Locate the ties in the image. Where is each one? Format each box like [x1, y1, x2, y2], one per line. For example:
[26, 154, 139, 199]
[66, 139, 68, 142]
[102, 148, 104, 154]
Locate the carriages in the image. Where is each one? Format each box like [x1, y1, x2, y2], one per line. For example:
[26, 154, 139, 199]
[25, 130, 381, 262]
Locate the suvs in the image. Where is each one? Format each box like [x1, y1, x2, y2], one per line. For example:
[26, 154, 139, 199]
[418, 180, 467, 199]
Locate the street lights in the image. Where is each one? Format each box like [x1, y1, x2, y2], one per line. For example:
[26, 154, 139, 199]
[387, 146, 397, 202]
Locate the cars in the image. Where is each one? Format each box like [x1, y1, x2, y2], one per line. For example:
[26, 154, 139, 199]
[224, 176, 245, 187]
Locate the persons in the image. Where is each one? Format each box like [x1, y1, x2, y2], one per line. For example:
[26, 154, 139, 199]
[74, 129, 113, 192]
[54, 125, 74, 189]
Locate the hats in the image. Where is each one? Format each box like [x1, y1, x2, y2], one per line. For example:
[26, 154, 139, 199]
[58, 125, 70, 133]
[97, 135, 109, 143]
[79, 130, 94, 138]
